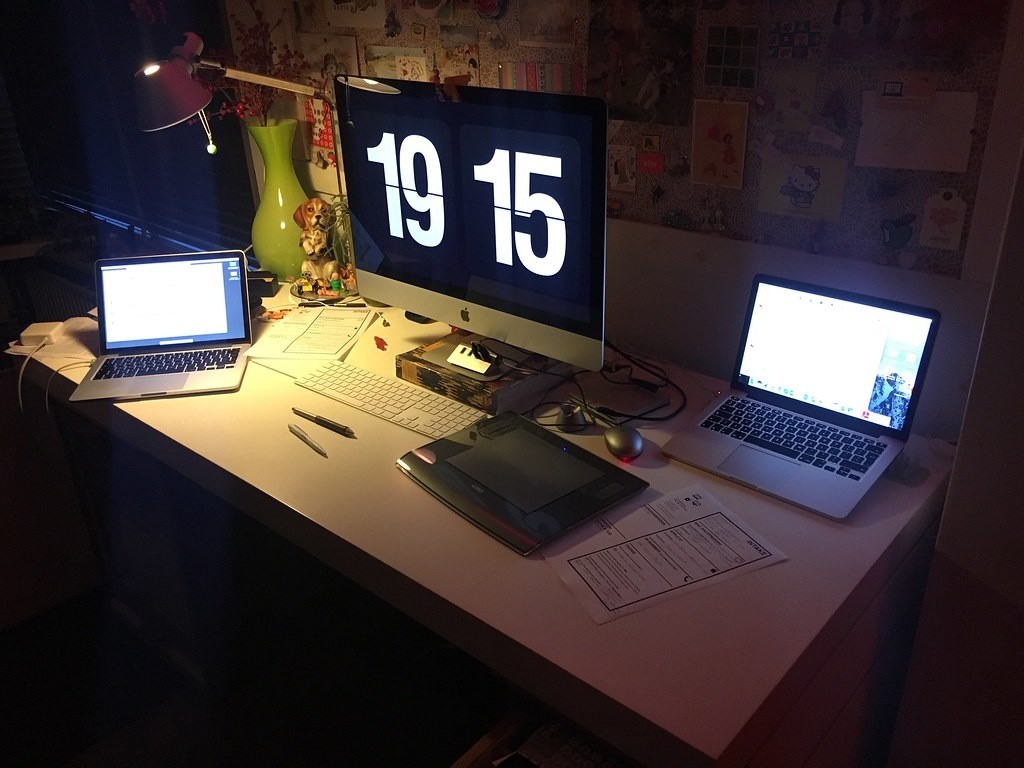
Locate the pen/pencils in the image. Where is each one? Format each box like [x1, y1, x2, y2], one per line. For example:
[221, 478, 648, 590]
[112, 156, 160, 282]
[293, 406, 356, 438]
[289, 422, 329, 460]
[299, 302, 365, 307]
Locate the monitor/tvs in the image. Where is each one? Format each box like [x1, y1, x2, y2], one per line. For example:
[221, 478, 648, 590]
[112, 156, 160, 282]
[334, 74, 609, 383]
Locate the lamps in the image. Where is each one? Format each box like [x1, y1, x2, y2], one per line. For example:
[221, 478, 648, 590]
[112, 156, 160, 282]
[133, 33, 354, 265]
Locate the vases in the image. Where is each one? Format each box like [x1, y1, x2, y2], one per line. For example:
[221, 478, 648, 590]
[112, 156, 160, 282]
[244, 119, 310, 281]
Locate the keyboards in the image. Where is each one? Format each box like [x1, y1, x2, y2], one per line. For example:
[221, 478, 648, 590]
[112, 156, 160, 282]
[295, 360, 487, 440]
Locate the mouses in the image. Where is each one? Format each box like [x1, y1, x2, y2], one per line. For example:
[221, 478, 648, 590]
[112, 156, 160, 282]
[603, 424, 643, 458]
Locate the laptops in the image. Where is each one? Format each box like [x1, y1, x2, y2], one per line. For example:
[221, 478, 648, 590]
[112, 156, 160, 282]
[658, 274, 939, 523]
[69, 249, 255, 404]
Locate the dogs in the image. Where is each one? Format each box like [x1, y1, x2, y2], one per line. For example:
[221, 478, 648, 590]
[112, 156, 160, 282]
[293, 197, 346, 290]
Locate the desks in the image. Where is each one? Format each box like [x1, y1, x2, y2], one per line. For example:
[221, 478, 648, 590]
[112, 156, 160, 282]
[16, 269, 958, 768]
